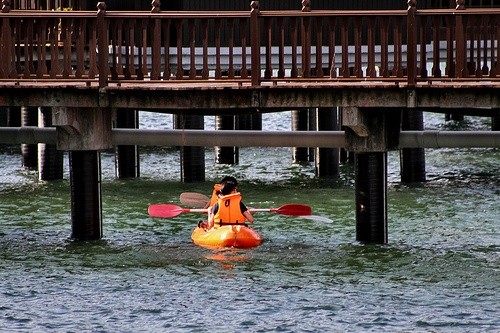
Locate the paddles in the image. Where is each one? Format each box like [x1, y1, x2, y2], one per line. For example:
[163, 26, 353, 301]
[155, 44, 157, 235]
[148, 203, 312, 219]
[180, 192, 334, 224]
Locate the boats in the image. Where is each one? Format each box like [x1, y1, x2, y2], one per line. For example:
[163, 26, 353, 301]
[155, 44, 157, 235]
[191, 221, 264, 251]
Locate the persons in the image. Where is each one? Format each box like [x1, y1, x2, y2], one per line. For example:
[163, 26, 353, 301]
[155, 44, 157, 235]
[198, 176, 257, 229]
